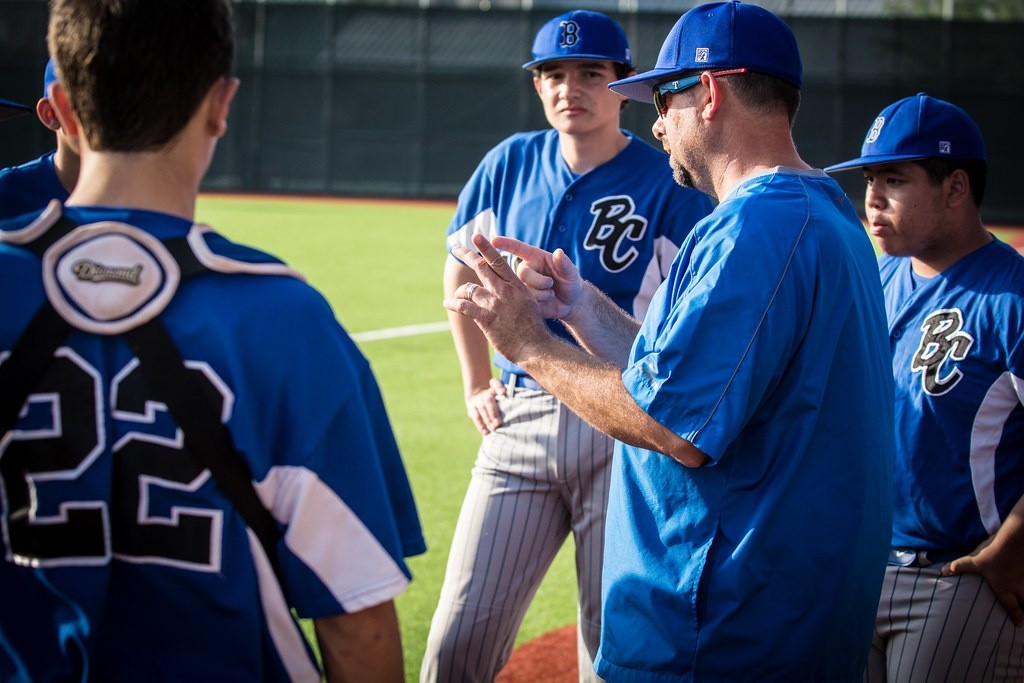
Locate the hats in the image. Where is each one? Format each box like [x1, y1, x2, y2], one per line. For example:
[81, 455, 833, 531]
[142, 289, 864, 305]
[607, 0, 804, 104]
[521, 9, 632, 72]
[821, 93, 988, 173]
[42, 57, 54, 103]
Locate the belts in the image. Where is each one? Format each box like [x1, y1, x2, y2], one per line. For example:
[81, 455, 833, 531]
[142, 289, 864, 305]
[887, 546, 981, 566]
[499, 370, 546, 391]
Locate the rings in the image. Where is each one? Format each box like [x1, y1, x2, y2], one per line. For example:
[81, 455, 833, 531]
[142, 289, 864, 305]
[468, 284, 479, 302]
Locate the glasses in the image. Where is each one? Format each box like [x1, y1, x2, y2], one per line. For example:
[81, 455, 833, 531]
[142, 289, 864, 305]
[652, 67, 745, 121]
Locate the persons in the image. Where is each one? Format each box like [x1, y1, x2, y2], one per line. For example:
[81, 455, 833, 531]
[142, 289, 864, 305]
[442, 0, 897, 683]
[0, 55, 82, 220]
[0, 0, 427, 683]
[416, 10, 720, 683]
[823, 94, 1024, 683]
